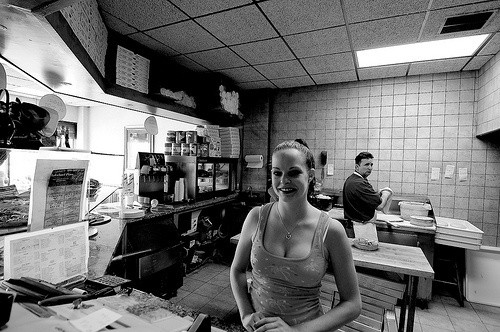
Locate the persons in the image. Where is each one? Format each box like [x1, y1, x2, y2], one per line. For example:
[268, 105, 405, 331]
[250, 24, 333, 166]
[59, 124, 70, 148]
[230, 137, 362, 332]
[342, 152, 392, 277]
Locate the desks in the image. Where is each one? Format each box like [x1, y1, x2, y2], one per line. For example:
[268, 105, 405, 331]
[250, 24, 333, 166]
[432, 244, 465, 303]
[334, 238, 435, 332]
[48, 290, 237, 332]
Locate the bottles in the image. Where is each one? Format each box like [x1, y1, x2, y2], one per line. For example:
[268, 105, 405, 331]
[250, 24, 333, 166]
[124, 188, 134, 209]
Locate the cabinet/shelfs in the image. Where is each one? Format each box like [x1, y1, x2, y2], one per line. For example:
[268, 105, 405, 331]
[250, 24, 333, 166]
[165, 154, 238, 201]
[101, 193, 237, 290]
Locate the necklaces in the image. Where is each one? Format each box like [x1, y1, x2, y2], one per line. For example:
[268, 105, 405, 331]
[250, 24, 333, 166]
[276, 200, 310, 240]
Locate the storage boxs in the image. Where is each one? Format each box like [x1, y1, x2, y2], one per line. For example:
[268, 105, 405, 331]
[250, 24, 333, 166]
[319, 271, 408, 332]
[433, 216, 485, 251]
[197, 124, 240, 158]
